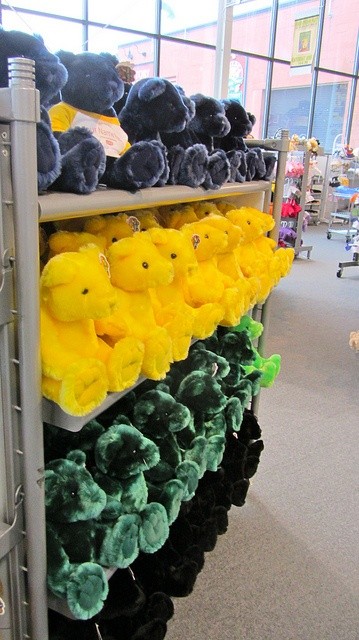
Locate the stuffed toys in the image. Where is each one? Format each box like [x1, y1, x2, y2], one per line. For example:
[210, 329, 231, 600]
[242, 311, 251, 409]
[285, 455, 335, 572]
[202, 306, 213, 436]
[0, 25, 276, 193]
[40, 200, 296, 415]
[44, 315, 282, 621]
[44, 315, 284, 621]
[48, 409, 264, 640]
[39, 199, 296, 417]
[0, 26, 277, 194]
[49, 410, 265, 640]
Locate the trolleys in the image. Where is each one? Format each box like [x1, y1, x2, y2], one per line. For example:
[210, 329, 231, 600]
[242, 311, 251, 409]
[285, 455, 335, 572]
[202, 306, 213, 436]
[327, 185, 359, 243]
[304, 174, 325, 226]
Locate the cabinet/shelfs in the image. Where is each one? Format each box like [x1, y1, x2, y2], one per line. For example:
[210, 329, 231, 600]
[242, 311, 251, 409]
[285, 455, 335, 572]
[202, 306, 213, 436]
[321, 137, 358, 238]
[266, 131, 316, 265]
[0, 52, 291, 640]
